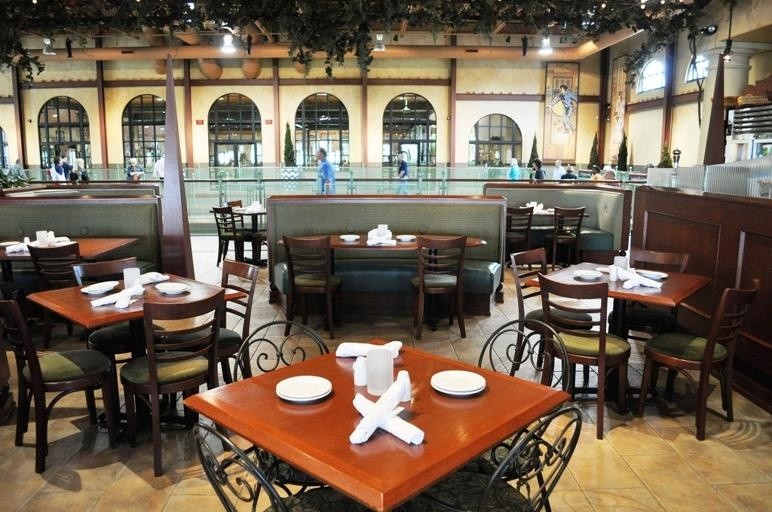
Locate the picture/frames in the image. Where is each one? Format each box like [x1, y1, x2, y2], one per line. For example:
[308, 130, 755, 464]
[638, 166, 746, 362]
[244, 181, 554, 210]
[540, 60, 581, 167]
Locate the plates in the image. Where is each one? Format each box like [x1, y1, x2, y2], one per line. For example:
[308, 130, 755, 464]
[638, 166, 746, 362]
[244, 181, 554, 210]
[340, 234, 360, 242]
[276, 375, 333, 403]
[395, 234, 416, 242]
[575, 270, 603, 280]
[431, 369, 487, 397]
[155, 282, 190, 294]
[636, 268, 669, 280]
[80, 281, 119, 294]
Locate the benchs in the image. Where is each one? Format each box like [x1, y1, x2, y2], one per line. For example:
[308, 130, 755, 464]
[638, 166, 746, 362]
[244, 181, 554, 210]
[17, 183, 162, 274]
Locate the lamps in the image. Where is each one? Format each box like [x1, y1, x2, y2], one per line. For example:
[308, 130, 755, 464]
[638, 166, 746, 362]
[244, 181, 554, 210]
[41, 37, 57, 57]
[700, 24, 719, 37]
[722, 1, 738, 64]
[538, 34, 555, 55]
[219, 34, 237, 54]
[372, 31, 388, 52]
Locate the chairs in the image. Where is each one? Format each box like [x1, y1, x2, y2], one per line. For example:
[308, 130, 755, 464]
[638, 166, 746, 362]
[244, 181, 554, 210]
[510, 247, 757, 441]
[3, 242, 258, 478]
[506, 205, 585, 272]
[182, 320, 582, 511]
[212, 199, 504, 340]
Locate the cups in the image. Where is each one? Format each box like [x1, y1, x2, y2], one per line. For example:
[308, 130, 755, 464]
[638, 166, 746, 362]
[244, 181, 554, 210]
[378, 225, 388, 229]
[221, 201, 263, 211]
[123, 268, 143, 289]
[614, 256, 628, 270]
[366, 349, 393, 395]
[526, 201, 554, 212]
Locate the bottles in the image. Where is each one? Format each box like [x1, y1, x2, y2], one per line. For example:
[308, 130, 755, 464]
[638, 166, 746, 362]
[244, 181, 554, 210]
[0, 230, 75, 253]
[608, 266, 618, 281]
[353, 357, 367, 386]
[397, 371, 411, 402]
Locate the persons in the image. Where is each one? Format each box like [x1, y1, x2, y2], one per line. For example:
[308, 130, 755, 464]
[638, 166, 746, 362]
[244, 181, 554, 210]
[50, 153, 89, 185]
[126, 158, 144, 181]
[548, 85, 577, 135]
[316, 148, 335, 195]
[509, 158, 520, 179]
[152, 156, 164, 180]
[591, 167, 601, 181]
[531, 159, 543, 184]
[604, 171, 620, 186]
[8, 159, 28, 179]
[552, 160, 564, 180]
[397, 154, 408, 195]
[560, 166, 577, 183]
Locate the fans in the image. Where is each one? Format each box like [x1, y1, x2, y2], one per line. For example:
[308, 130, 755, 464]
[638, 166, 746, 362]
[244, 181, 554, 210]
[386, 97, 429, 114]
